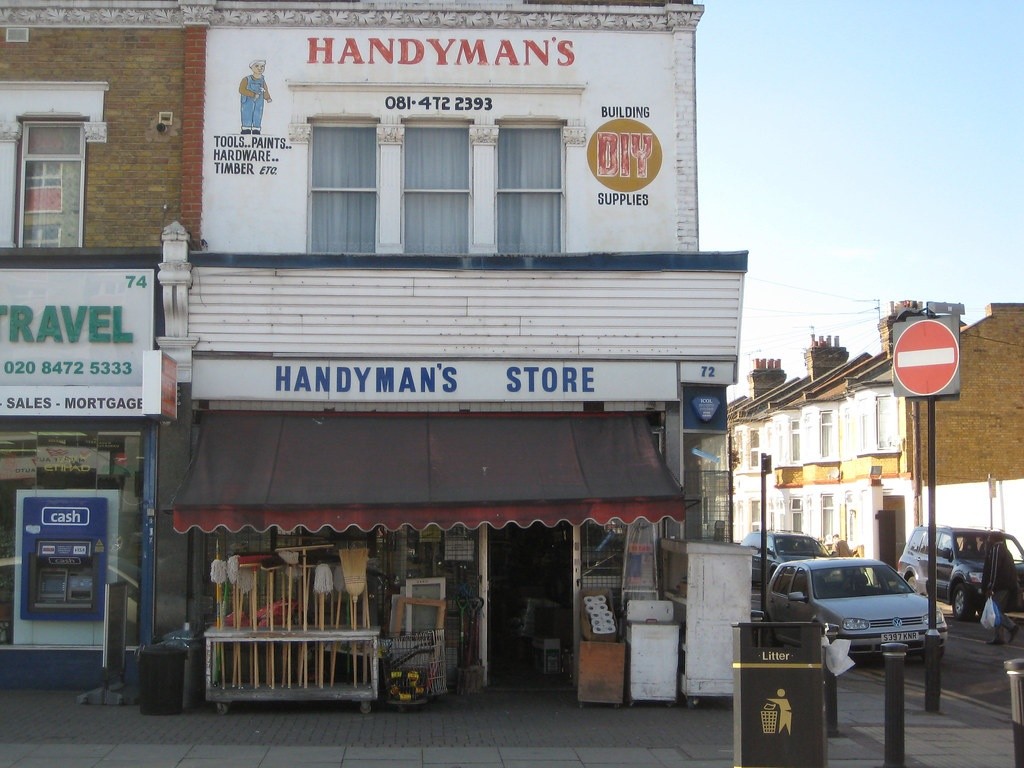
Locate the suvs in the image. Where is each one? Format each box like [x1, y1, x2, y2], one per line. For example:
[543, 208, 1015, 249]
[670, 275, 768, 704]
[897, 524, 1024, 620]
[740, 530, 830, 590]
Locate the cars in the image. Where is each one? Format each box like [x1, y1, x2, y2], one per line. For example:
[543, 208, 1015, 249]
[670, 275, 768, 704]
[766, 557, 948, 665]
[0, 552, 211, 652]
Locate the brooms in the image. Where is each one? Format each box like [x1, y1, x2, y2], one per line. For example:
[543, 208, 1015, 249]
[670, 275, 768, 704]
[238, 535, 369, 689]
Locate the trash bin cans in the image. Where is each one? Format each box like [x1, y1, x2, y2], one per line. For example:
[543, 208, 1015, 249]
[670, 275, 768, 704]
[732, 620, 829, 768]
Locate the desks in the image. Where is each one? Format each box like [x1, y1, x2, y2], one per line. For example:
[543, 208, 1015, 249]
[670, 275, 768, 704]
[533, 635, 561, 675]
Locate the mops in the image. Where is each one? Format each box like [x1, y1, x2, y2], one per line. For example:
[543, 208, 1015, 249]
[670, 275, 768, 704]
[211, 552, 346, 689]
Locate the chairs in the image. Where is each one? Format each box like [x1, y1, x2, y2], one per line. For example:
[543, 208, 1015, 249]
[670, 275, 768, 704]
[846, 574, 873, 597]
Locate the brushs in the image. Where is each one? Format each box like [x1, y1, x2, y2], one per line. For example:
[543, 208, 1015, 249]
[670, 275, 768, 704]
[237, 551, 342, 689]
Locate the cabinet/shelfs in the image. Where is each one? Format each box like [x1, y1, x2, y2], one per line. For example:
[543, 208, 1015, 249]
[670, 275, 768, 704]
[661, 538, 758, 708]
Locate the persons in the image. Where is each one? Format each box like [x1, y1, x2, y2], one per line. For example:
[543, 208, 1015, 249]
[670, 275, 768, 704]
[980, 532, 1019, 645]
[833, 534, 852, 557]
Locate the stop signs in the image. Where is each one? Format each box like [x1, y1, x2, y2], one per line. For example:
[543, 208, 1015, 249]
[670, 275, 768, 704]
[891, 315, 960, 398]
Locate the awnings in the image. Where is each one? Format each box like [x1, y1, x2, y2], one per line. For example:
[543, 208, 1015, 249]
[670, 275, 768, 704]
[166, 410, 687, 534]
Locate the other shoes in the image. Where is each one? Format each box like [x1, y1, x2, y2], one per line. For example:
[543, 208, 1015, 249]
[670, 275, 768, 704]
[985, 637, 1005, 645]
[1008, 625, 1019, 642]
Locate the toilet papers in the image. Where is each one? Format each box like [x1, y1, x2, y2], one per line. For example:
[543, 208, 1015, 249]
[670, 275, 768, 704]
[584, 595, 616, 634]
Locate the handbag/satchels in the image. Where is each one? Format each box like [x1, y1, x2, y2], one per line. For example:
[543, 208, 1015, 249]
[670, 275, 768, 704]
[980, 595, 1001, 629]
